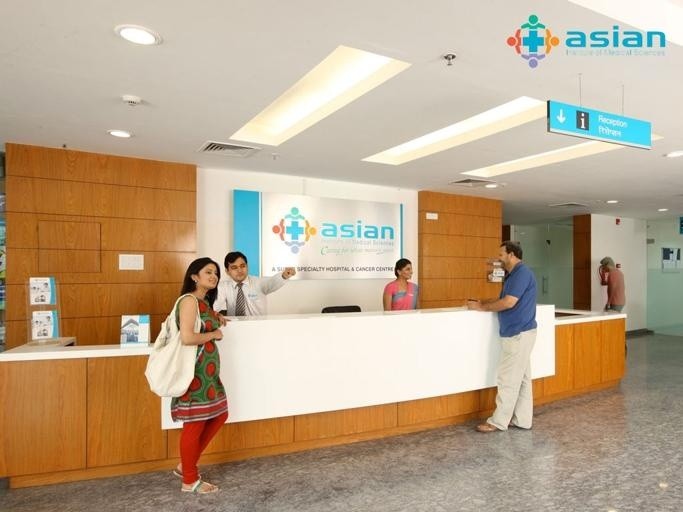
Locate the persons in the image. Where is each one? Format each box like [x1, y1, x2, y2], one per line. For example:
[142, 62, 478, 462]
[42, 316, 52, 325]
[598, 254, 628, 358]
[465, 239, 539, 434]
[211, 250, 296, 317]
[41, 282, 49, 292]
[382, 258, 419, 311]
[169, 256, 232, 494]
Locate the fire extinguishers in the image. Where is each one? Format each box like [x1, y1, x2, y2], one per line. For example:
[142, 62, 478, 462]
[599, 266, 609, 285]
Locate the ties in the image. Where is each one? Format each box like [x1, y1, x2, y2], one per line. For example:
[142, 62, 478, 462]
[236, 283, 245, 316]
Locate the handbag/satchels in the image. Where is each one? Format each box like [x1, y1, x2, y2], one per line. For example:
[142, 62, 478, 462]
[144, 294, 202, 397]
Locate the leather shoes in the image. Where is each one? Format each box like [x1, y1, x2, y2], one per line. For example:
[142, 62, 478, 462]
[475, 421, 515, 432]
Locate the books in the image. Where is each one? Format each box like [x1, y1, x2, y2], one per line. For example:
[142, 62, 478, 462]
[31, 310, 59, 339]
[29, 278, 56, 305]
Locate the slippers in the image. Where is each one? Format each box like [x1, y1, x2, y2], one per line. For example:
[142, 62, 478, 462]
[172, 466, 218, 494]
